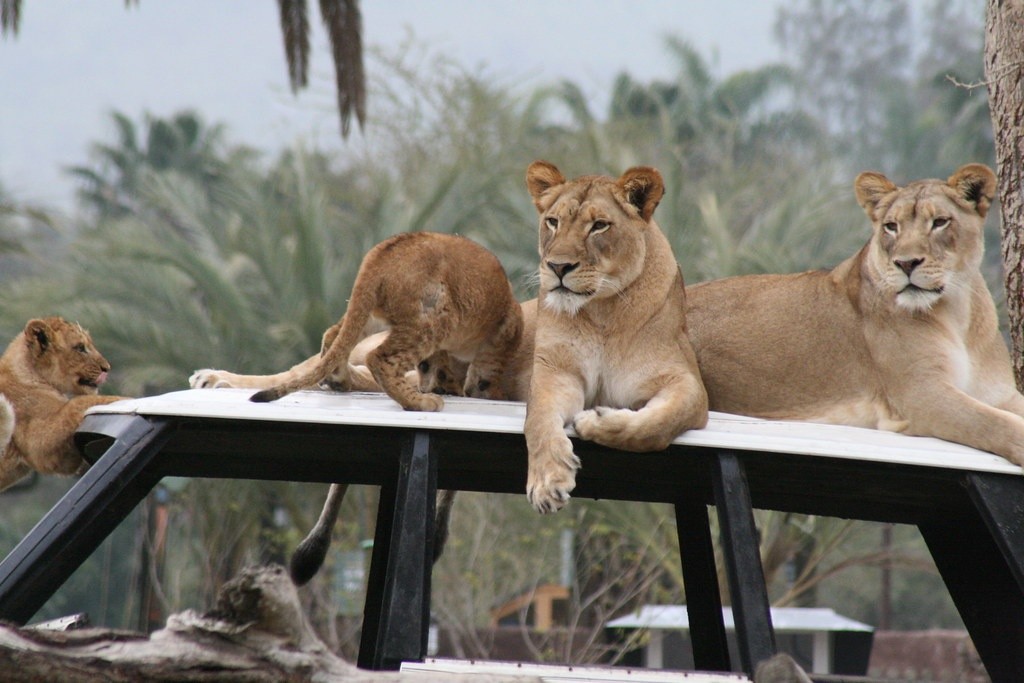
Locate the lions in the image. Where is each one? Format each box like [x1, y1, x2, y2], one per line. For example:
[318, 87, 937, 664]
[0, 315, 134, 490]
[186, 159, 1024, 520]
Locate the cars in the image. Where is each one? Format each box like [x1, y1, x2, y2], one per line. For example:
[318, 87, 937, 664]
[0, 389, 1024, 683]
